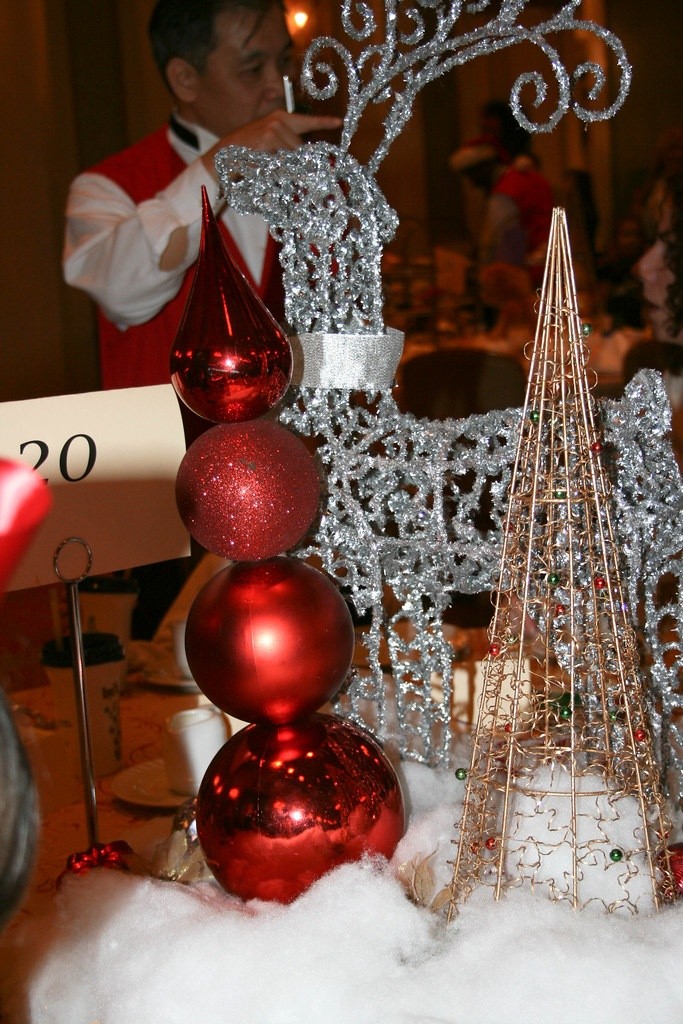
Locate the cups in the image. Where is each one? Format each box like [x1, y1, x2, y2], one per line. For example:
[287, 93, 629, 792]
[159, 704, 229, 796]
[40, 630, 124, 778]
[75, 576, 141, 694]
[173, 618, 193, 680]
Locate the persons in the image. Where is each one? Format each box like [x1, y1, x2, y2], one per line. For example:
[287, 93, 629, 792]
[59, 0, 343, 639]
[450, 98, 683, 474]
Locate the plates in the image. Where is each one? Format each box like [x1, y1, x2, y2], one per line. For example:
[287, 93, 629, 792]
[148, 672, 200, 694]
[109, 757, 190, 806]
